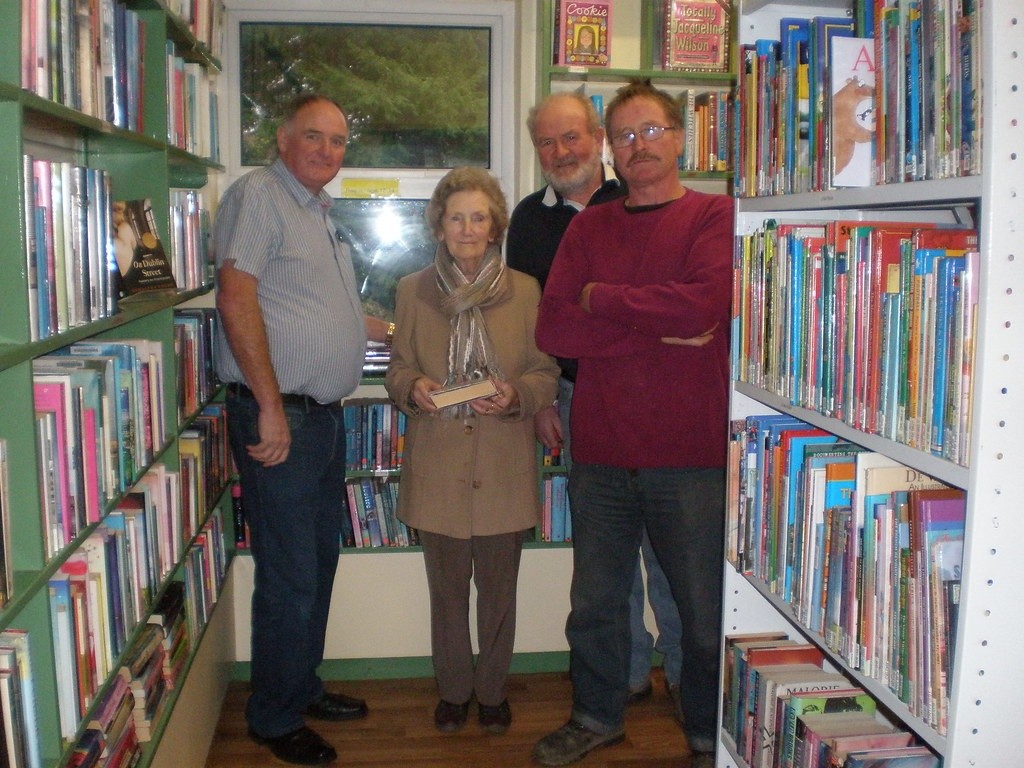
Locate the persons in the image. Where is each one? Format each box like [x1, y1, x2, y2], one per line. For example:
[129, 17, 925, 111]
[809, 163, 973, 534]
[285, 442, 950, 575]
[534, 81, 736, 768]
[506, 93, 685, 725]
[213, 93, 395, 765]
[385, 166, 564, 737]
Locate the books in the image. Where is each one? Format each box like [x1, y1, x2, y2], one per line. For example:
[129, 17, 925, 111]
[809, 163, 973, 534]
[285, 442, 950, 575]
[0, 1, 986, 768]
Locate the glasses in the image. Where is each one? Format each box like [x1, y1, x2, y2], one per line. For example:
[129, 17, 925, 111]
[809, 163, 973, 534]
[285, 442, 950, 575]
[608, 126, 675, 149]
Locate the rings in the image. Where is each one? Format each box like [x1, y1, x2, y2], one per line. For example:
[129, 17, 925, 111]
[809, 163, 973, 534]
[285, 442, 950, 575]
[491, 404, 494, 411]
[484, 409, 489, 417]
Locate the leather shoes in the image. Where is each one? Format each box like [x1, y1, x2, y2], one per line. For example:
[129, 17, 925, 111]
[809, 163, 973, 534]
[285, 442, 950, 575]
[247, 720, 337, 766]
[302, 689, 367, 721]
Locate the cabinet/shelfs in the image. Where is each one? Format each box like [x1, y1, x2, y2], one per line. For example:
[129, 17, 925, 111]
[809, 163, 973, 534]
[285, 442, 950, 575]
[533, 0, 738, 191]
[0, 0, 237, 768]
[715, 0, 1024, 768]
[232, 367, 574, 556]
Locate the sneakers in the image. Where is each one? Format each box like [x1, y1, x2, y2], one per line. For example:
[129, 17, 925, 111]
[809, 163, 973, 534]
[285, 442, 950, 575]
[433, 696, 473, 734]
[689, 749, 716, 767]
[477, 699, 513, 734]
[531, 715, 627, 767]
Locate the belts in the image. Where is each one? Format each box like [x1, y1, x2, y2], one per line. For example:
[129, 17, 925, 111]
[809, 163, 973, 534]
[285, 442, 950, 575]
[227, 382, 328, 409]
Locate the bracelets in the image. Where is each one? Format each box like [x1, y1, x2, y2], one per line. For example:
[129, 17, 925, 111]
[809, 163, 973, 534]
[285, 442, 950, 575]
[386, 323, 395, 345]
